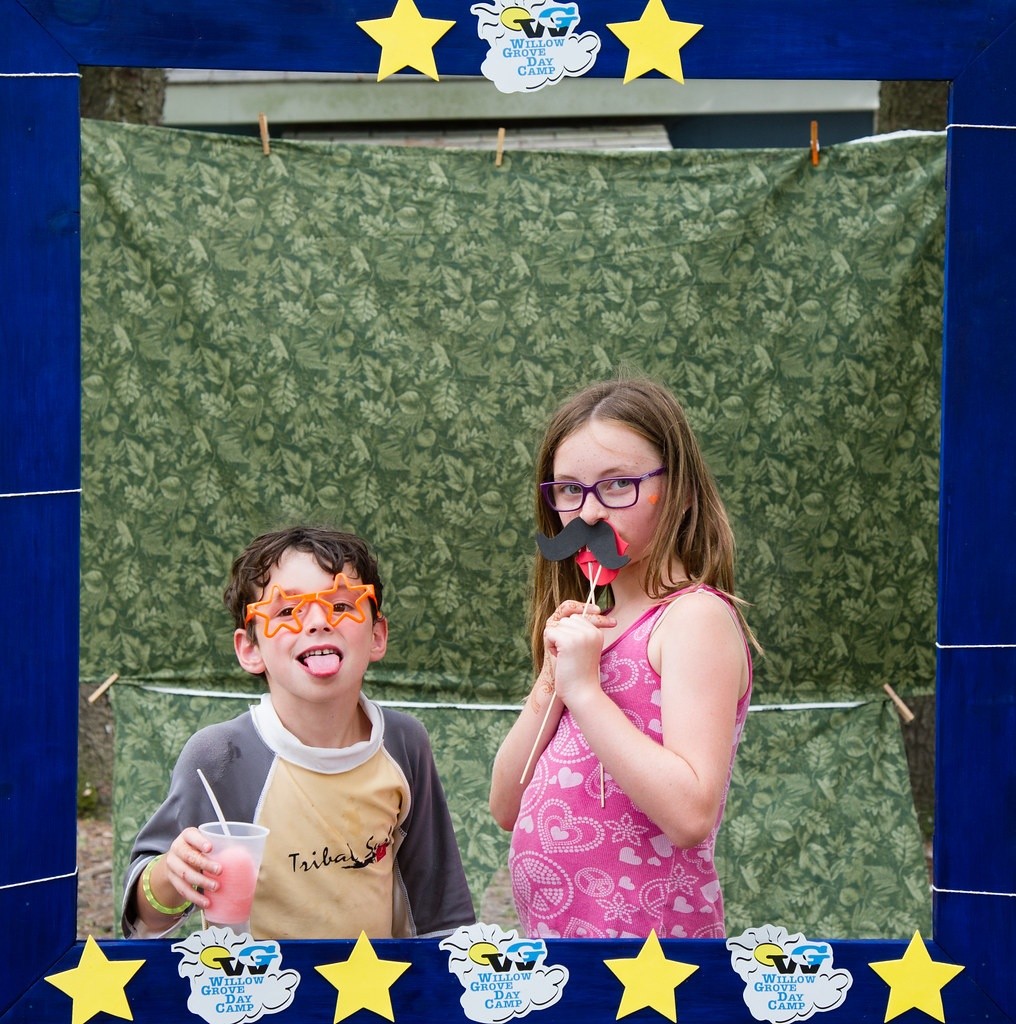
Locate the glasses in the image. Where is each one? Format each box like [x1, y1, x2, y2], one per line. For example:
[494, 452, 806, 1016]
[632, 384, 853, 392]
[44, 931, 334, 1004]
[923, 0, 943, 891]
[540, 467, 668, 512]
[246, 572, 380, 639]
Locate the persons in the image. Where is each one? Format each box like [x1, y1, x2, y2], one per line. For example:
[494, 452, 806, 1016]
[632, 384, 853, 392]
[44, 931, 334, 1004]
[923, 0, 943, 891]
[119, 527, 476, 939]
[489, 381, 765, 939]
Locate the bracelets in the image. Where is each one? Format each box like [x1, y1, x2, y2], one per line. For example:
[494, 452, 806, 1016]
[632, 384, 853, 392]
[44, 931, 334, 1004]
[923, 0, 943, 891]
[142, 853, 199, 915]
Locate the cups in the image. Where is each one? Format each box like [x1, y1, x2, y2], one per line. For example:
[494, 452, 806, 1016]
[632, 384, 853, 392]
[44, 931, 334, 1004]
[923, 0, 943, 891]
[198, 821, 270, 924]
